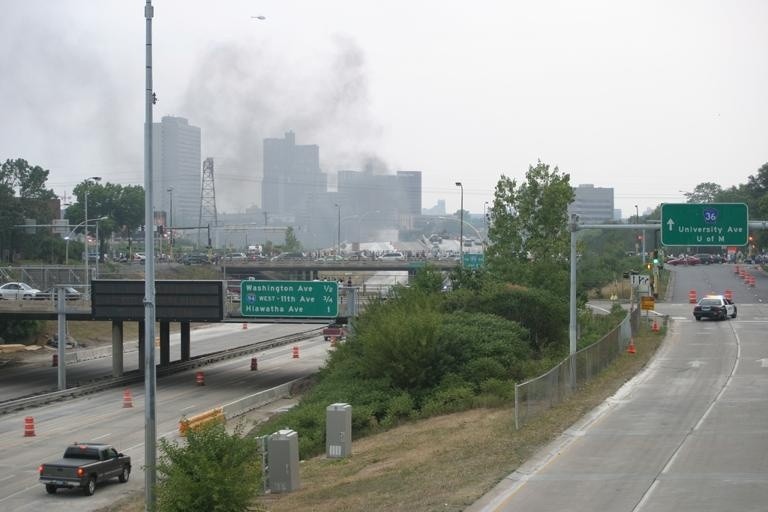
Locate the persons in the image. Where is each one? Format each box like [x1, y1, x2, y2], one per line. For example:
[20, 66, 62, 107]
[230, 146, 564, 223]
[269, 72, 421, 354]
[313, 276, 352, 287]
[670, 250, 768, 266]
[116, 247, 459, 264]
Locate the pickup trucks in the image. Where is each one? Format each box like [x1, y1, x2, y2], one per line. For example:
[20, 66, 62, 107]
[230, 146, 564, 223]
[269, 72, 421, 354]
[39, 443, 131, 497]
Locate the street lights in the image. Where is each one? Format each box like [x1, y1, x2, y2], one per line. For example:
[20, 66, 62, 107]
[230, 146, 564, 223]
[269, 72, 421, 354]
[455, 182, 463, 253]
[483, 201, 488, 235]
[166, 189, 172, 251]
[634, 205, 638, 223]
[65, 176, 108, 265]
[333, 203, 340, 251]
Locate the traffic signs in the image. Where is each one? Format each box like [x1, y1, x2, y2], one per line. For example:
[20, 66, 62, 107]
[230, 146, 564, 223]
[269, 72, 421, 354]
[660, 203, 748, 246]
[239, 280, 338, 317]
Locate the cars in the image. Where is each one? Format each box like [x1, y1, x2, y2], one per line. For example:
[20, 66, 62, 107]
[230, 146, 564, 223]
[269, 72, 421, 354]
[625, 250, 634, 257]
[222, 252, 248, 262]
[439, 253, 464, 262]
[134, 252, 145, 264]
[270, 251, 306, 264]
[0, 282, 79, 301]
[227, 290, 239, 303]
[325, 255, 344, 260]
[323, 324, 347, 341]
[666, 253, 767, 266]
[692, 294, 736, 321]
[375, 251, 406, 261]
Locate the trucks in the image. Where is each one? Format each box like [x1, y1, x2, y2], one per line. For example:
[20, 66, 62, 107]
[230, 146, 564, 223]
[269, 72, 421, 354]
[432, 233, 449, 249]
[463, 236, 480, 247]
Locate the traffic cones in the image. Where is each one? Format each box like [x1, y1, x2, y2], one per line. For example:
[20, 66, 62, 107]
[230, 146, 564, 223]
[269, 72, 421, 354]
[650, 317, 659, 332]
[626, 336, 635, 353]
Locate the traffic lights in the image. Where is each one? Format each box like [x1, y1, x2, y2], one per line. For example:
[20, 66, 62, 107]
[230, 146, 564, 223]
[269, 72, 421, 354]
[652, 250, 658, 264]
[637, 235, 642, 240]
